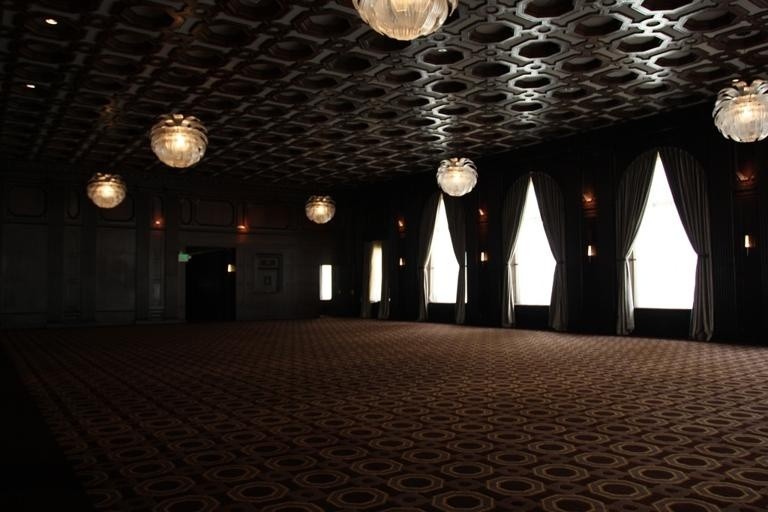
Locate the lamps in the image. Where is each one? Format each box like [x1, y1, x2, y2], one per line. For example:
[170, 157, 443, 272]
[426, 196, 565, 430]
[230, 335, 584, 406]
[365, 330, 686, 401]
[711, 27, 768, 143]
[434, 146, 481, 198]
[84, 112, 210, 209]
[303, 182, 337, 227]
[350, 0, 460, 44]
